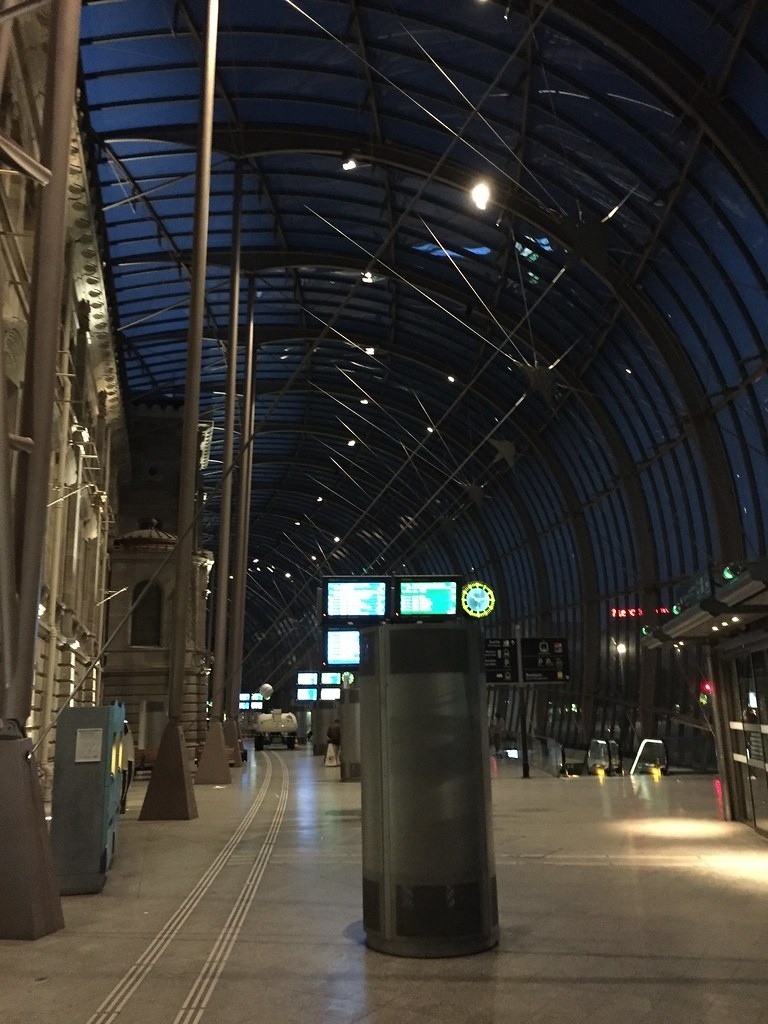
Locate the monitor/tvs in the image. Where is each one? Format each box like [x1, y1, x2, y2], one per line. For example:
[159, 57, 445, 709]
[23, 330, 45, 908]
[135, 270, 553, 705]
[239, 693, 263, 710]
[297, 571, 465, 702]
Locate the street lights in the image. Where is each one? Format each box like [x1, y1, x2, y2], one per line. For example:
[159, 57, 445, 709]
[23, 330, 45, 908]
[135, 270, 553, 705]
[193, 143, 492, 789]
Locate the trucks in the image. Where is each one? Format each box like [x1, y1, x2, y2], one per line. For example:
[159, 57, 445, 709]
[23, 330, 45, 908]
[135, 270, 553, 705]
[252, 708, 297, 751]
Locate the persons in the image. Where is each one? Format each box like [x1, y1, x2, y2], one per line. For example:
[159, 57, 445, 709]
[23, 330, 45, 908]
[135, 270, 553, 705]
[324, 719, 340, 763]
